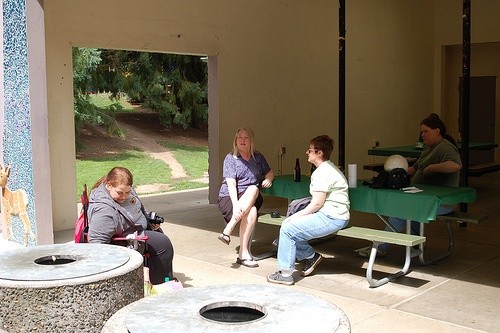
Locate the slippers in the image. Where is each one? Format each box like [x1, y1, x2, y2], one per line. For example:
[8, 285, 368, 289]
[236, 258, 260, 267]
[218, 233, 230, 245]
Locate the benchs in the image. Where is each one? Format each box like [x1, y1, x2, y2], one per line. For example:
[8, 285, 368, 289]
[432, 210, 492, 253]
[468, 162, 500, 176]
[363, 159, 417, 171]
[249, 213, 426, 286]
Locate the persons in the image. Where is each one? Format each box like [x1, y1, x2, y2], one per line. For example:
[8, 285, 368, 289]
[86, 168, 178, 287]
[218, 128, 276, 268]
[265, 134, 352, 287]
[359, 118, 462, 257]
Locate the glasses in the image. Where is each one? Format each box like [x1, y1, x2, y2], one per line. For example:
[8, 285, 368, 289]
[309, 148, 319, 153]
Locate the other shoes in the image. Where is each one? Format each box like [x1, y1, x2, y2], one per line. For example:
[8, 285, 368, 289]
[359, 247, 386, 259]
[410, 248, 421, 262]
[266, 270, 295, 285]
[300, 252, 323, 277]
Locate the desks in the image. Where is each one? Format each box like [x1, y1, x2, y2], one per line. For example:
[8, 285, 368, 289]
[260, 172, 480, 270]
[367, 142, 498, 158]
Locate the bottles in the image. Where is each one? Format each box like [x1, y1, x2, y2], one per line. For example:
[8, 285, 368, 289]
[311, 163, 317, 175]
[294, 158, 301, 182]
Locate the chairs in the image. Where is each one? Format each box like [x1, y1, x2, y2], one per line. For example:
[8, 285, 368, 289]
[79, 183, 153, 296]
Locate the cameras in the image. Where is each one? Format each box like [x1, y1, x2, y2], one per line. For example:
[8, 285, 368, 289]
[147, 211, 164, 230]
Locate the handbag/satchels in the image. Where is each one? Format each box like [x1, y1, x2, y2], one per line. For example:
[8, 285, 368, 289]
[369, 168, 412, 189]
